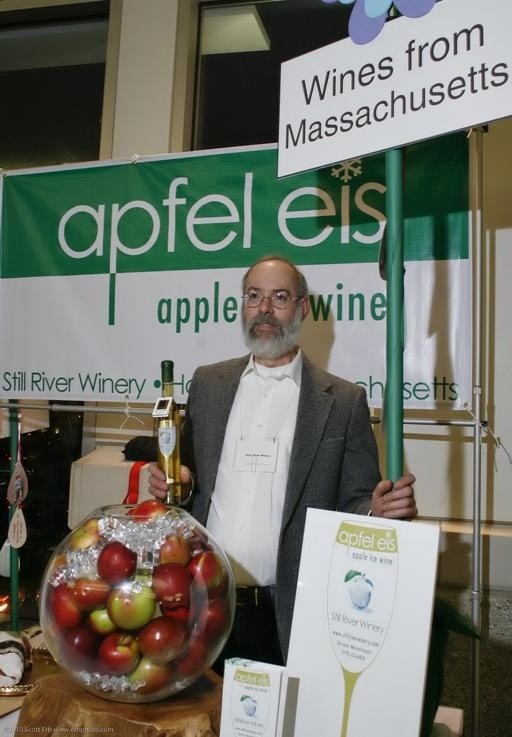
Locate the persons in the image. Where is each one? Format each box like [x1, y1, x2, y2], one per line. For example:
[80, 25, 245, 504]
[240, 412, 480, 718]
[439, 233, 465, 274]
[146, 257, 419, 663]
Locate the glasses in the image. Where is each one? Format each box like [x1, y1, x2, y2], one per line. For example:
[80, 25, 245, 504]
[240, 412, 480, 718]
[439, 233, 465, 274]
[240, 288, 301, 310]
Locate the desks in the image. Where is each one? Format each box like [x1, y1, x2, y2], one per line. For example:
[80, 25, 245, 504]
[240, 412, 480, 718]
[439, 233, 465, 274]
[67, 445, 159, 531]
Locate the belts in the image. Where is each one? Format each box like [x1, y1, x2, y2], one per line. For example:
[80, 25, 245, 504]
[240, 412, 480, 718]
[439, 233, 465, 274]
[228, 584, 276, 606]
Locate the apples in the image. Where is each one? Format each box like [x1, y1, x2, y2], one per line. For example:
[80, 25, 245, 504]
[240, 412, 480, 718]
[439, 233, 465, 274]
[50, 498, 230, 695]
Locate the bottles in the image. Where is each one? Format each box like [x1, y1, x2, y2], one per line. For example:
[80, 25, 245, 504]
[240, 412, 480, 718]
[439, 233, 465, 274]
[156, 362, 182, 504]
